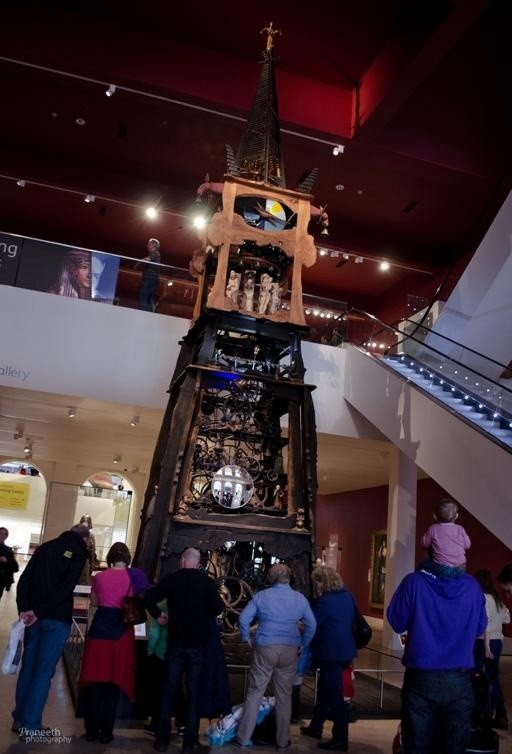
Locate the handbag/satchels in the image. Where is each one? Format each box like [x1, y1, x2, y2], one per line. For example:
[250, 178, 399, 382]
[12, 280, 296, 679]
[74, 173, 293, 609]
[121, 595, 148, 625]
[354, 607, 373, 650]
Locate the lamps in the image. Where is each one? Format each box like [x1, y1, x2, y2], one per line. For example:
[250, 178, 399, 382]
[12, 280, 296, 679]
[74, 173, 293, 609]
[1, 56, 346, 159]
[120, 269, 367, 320]
[0, 174, 433, 275]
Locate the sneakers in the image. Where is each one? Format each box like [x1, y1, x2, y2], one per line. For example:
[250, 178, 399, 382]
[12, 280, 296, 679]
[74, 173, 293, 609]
[84, 730, 115, 744]
[232, 739, 248, 752]
[144, 725, 157, 736]
[317, 738, 349, 753]
[11, 721, 56, 743]
[470, 716, 507, 730]
[278, 739, 291, 751]
[153, 739, 168, 754]
[177, 726, 186, 735]
[299, 725, 323, 741]
[183, 742, 212, 753]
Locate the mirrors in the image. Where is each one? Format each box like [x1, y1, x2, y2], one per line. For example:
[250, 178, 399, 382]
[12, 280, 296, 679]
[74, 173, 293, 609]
[211, 465, 254, 509]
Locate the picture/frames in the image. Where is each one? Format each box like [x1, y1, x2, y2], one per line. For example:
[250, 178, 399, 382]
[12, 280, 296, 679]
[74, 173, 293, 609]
[368, 529, 388, 610]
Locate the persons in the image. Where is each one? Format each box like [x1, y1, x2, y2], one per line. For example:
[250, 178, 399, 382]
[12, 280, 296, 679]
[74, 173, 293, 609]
[91, 253, 107, 299]
[319, 335, 331, 346]
[49, 249, 91, 301]
[133, 238, 162, 313]
[225, 270, 284, 316]
[4, 497, 511, 753]
[273, 484, 285, 511]
[213, 487, 252, 509]
[329, 326, 341, 347]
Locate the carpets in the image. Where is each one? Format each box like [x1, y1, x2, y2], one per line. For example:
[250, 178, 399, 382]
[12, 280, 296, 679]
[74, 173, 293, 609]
[61, 638, 401, 721]
